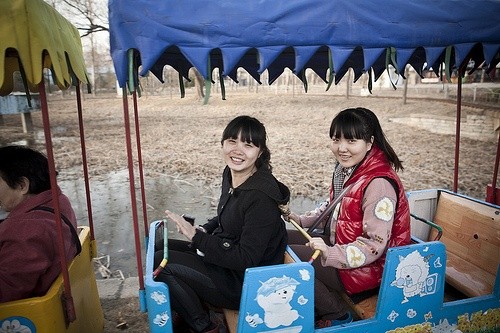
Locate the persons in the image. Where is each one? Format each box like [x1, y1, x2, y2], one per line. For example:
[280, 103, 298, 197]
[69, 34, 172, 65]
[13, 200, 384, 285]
[154, 116, 291, 333]
[0, 145, 78, 303]
[284, 107, 412, 329]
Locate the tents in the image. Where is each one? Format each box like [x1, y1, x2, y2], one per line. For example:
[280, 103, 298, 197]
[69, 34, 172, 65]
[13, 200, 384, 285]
[0, 0, 96, 297]
[107, 0, 500, 291]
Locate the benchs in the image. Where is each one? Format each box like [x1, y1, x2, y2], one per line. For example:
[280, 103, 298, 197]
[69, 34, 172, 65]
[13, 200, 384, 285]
[0, 225, 104, 333]
[223, 244, 315, 333]
[334, 237, 445, 320]
[433, 191, 500, 319]
